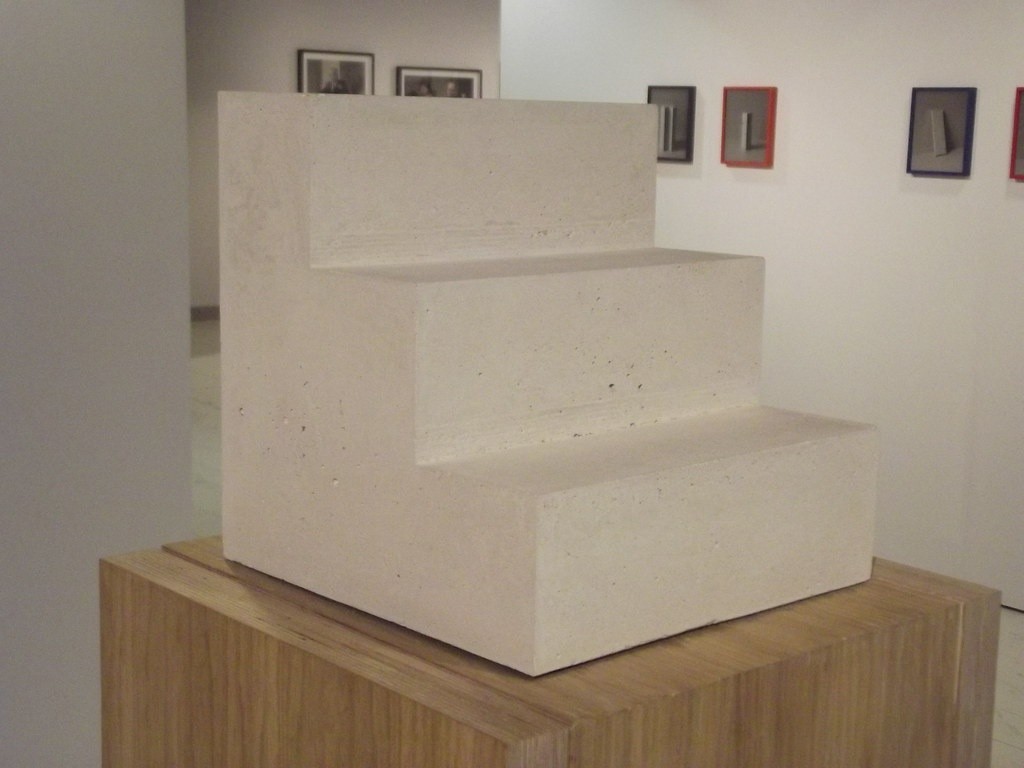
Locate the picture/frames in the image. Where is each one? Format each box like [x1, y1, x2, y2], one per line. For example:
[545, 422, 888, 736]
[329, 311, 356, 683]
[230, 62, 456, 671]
[646, 84, 697, 161]
[718, 85, 778, 168]
[1007, 87, 1024, 180]
[396, 66, 482, 99]
[905, 85, 977, 176]
[299, 47, 374, 96]
